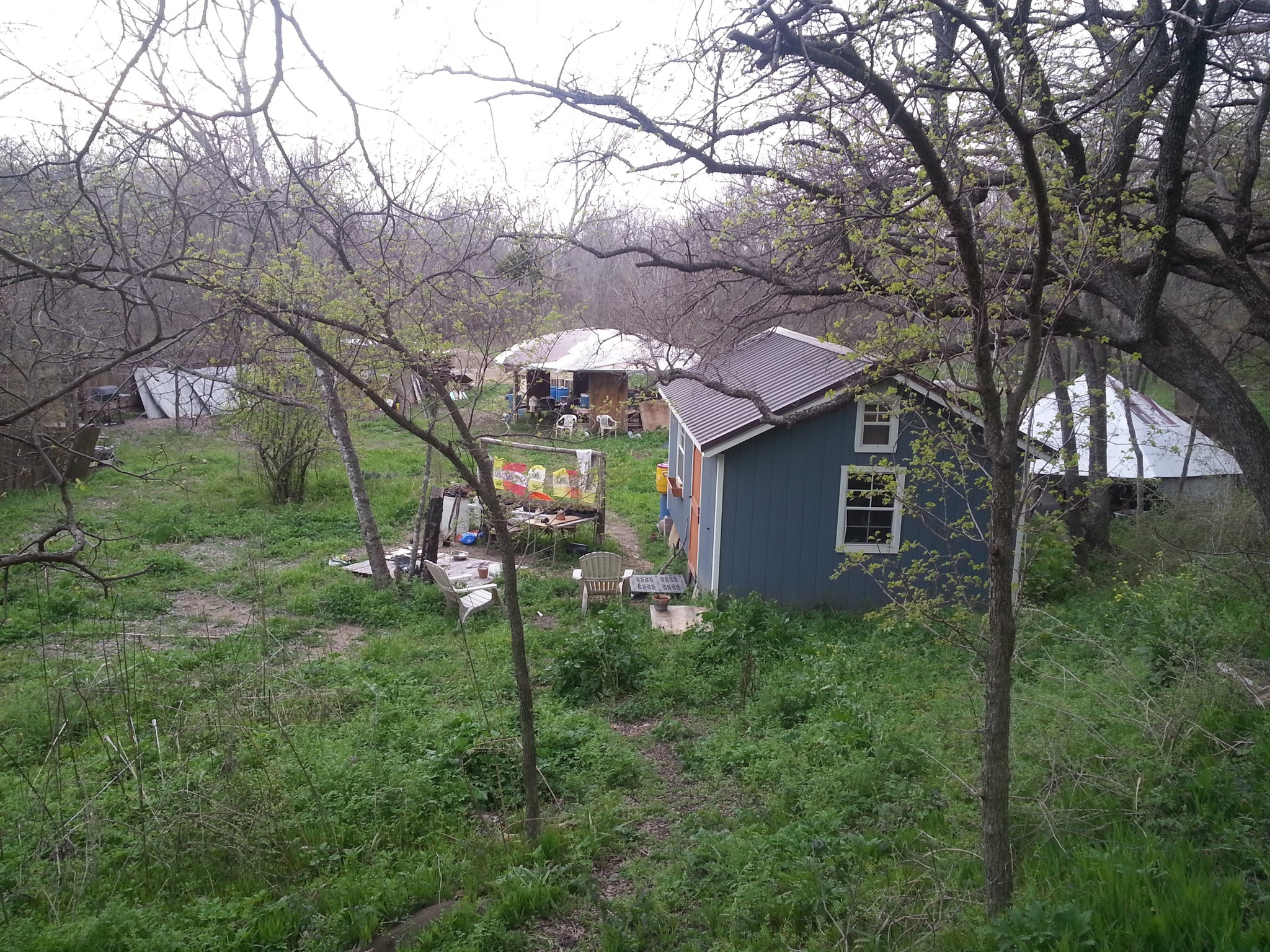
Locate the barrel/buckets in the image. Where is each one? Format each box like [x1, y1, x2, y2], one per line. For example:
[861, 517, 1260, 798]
[550, 386, 569, 401]
[580, 393, 589, 408]
[509, 394, 522, 409]
[655, 461, 668, 521]
[458, 532, 475, 544]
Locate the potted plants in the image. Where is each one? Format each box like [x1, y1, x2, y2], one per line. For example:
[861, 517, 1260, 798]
[522, 501, 601, 518]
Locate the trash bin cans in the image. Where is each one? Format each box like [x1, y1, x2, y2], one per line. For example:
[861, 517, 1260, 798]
[508, 393, 522, 411]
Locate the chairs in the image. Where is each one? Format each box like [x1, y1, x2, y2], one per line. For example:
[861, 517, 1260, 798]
[555, 414, 577, 440]
[486, 520, 524, 555]
[424, 559, 507, 628]
[596, 415, 617, 438]
[572, 551, 633, 617]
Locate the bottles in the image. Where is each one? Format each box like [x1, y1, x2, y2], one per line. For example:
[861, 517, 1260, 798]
[628, 430, 633, 438]
[633, 433, 642, 439]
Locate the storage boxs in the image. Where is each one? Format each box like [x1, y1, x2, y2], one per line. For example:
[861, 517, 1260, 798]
[580, 393, 589, 408]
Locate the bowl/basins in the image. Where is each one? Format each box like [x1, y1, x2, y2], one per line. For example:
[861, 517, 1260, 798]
[451, 550, 469, 560]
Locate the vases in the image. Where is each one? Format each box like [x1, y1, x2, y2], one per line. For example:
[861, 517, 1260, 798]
[478, 567, 488, 579]
[650, 534, 658, 543]
[555, 511, 565, 520]
[652, 594, 670, 611]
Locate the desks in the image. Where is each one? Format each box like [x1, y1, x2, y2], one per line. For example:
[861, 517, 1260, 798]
[467, 503, 599, 556]
[525, 410, 540, 425]
